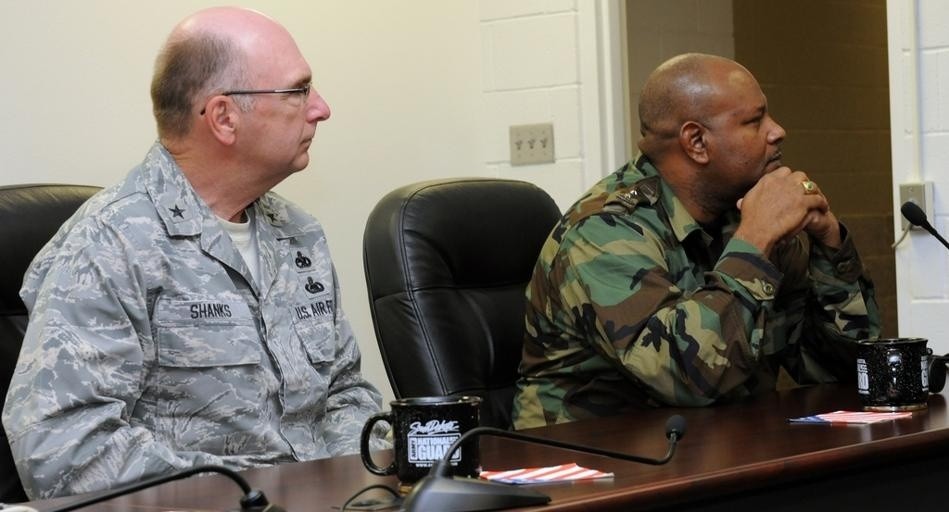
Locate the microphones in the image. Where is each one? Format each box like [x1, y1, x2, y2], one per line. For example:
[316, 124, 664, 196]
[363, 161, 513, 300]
[47, 464, 284, 512]
[900, 201, 949, 363]
[400, 415, 685, 512]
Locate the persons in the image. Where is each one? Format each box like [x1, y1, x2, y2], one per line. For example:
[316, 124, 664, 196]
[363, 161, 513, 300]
[0, 5, 396, 500]
[510, 51, 883, 431]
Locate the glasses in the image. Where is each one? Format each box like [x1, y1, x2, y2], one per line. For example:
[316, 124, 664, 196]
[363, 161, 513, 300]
[200, 79, 310, 117]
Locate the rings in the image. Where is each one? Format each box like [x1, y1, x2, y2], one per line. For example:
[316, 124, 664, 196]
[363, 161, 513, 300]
[799, 177, 817, 191]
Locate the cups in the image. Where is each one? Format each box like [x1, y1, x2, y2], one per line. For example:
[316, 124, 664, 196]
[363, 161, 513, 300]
[854, 337, 930, 412]
[360, 396, 483, 485]
